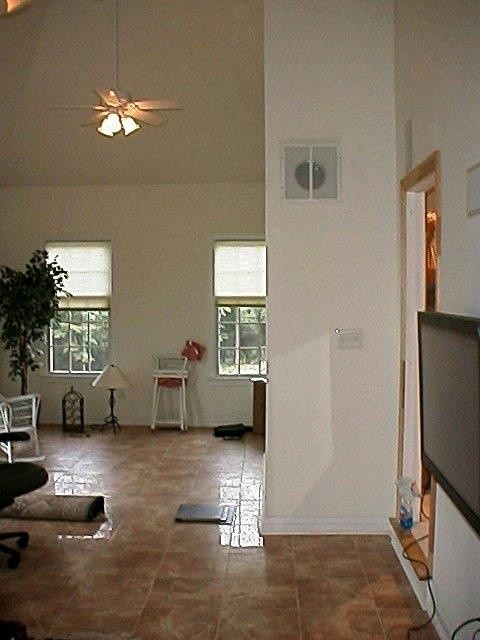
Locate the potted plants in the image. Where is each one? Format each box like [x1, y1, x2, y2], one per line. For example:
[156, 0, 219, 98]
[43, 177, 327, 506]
[0, 248, 75, 430]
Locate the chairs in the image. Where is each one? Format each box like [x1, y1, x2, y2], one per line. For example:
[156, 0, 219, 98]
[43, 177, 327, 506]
[1, 432, 49, 569]
[0, 393, 48, 464]
[150, 356, 190, 431]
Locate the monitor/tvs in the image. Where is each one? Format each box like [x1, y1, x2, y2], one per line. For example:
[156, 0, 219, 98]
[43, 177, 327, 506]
[417, 311, 480, 542]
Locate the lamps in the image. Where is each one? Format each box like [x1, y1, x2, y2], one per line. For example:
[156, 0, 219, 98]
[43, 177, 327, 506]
[0, 0, 32, 15]
[91, 363, 130, 435]
[97, 112, 141, 138]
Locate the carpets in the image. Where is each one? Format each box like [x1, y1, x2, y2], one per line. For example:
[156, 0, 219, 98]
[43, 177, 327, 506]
[1, 493, 108, 536]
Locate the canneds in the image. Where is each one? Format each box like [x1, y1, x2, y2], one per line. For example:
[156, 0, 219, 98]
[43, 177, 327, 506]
[399, 506, 413, 528]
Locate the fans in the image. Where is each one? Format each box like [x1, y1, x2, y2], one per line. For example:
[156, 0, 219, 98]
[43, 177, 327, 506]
[53, 0, 184, 127]
